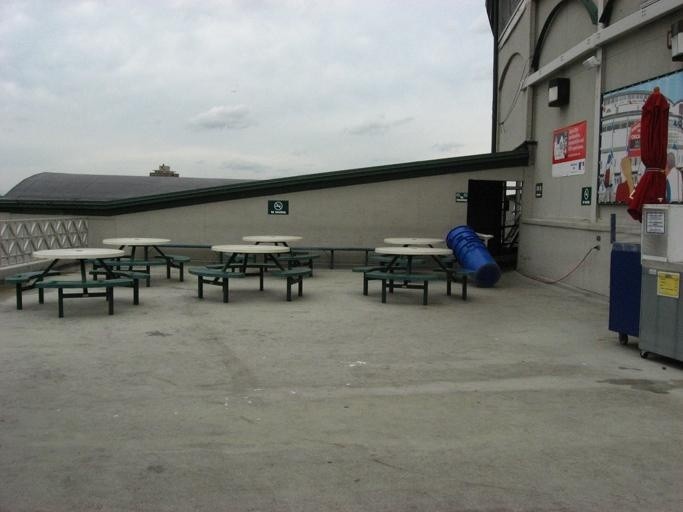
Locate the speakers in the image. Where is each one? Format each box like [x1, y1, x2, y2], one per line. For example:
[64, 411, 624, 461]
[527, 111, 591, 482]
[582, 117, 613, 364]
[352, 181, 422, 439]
[548, 78, 570, 107]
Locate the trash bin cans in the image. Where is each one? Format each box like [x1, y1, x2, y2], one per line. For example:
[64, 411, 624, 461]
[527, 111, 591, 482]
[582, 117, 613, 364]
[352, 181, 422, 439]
[608, 242, 642, 345]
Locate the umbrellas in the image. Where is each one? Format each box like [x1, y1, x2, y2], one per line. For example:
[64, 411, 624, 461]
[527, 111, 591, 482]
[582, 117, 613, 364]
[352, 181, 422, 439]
[626, 86, 670, 223]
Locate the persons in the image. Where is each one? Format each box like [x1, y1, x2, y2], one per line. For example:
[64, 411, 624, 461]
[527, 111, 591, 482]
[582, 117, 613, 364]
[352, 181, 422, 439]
[553, 135, 566, 161]
[665, 152, 682, 204]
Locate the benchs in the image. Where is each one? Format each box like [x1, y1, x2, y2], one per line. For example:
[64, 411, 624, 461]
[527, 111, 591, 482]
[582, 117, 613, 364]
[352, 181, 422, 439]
[189, 236, 321, 304]
[352, 237, 474, 305]
[92, 237, 190, 287]
[0, 247, 149, 320]
